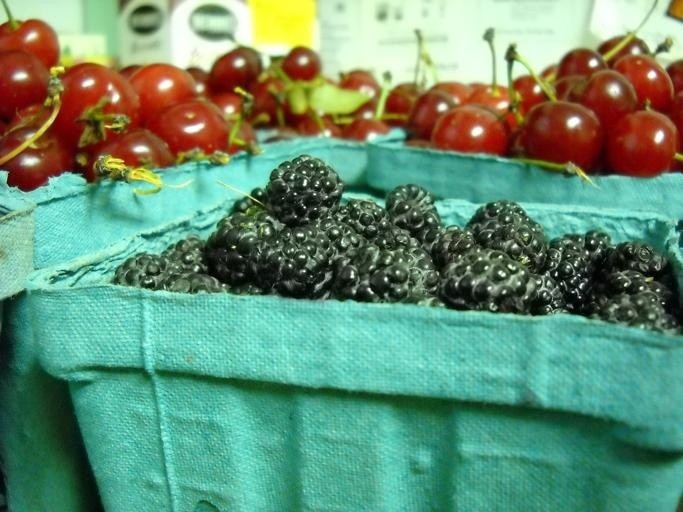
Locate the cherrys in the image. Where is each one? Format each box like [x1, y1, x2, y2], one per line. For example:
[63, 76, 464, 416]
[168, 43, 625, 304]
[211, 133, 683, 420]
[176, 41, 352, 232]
[0, 17, 683, 194]
